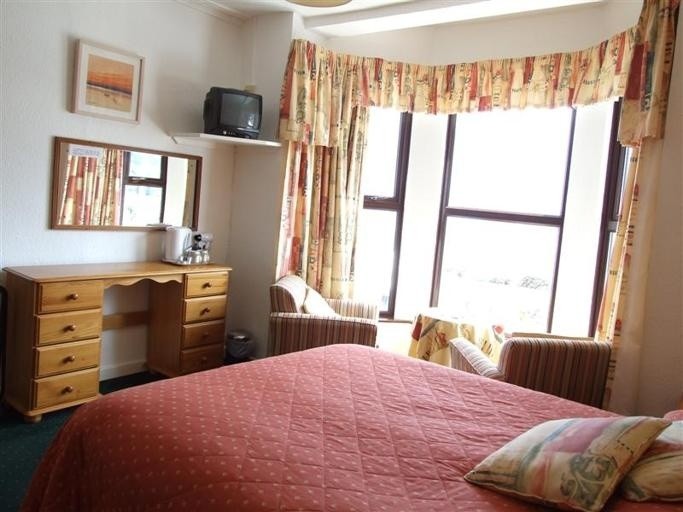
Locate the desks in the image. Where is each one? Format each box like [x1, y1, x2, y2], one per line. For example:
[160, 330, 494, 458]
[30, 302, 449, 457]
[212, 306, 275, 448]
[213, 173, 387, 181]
[2, 260, 233, 423]
[409, 308, 507, 367]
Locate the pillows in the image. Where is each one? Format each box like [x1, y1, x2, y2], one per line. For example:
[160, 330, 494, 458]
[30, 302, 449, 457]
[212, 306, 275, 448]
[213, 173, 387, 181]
[302, 287, 338, 314]
[622, 420, 683, 504]
[463, 416, 672, 511]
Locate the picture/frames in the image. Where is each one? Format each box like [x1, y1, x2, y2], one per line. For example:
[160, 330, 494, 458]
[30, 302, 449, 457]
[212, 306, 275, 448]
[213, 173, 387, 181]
[71, 38, 146, 123]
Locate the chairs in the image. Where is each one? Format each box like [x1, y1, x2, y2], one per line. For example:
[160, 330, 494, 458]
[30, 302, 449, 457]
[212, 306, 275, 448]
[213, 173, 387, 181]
[269, 273, 378, 358]
[450, 332, 611, 412]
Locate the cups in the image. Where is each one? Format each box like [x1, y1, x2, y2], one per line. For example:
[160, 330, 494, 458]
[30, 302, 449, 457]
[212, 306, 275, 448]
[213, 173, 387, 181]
[201, 249, 210, 264]
[193, 251, 202, 263]
[187, 251, 192, 264]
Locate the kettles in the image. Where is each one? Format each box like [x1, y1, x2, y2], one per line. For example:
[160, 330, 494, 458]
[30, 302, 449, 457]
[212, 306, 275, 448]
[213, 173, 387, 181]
[164, 226, 192, 261]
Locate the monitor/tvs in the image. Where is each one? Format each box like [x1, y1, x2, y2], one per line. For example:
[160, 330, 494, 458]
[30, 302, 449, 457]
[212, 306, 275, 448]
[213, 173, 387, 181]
[202, 85, 262, 139]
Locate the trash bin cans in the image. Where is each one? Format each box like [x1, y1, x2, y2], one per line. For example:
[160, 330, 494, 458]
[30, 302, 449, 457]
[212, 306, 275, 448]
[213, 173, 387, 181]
[226, 328, 255, 364]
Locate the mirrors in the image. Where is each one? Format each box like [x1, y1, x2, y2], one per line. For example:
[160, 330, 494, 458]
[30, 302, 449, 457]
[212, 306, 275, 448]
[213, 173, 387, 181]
[51, 135, 202, 230]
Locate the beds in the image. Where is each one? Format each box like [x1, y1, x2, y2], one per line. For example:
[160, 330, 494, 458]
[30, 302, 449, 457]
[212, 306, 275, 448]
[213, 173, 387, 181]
[22, 342, 683, 512]
